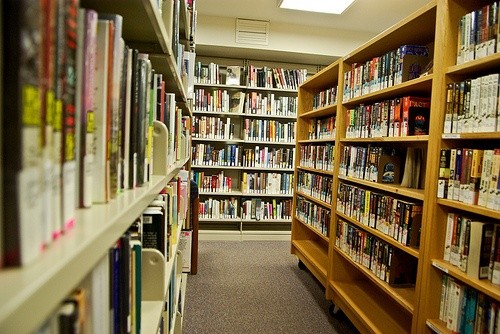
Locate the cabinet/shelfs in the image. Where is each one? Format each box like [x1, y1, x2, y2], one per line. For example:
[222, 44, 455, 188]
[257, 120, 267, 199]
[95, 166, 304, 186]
[0, 0, 500, 334]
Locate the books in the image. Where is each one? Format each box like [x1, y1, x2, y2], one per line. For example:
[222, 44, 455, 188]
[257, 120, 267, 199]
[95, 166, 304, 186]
[0, 0, 500, 334]
